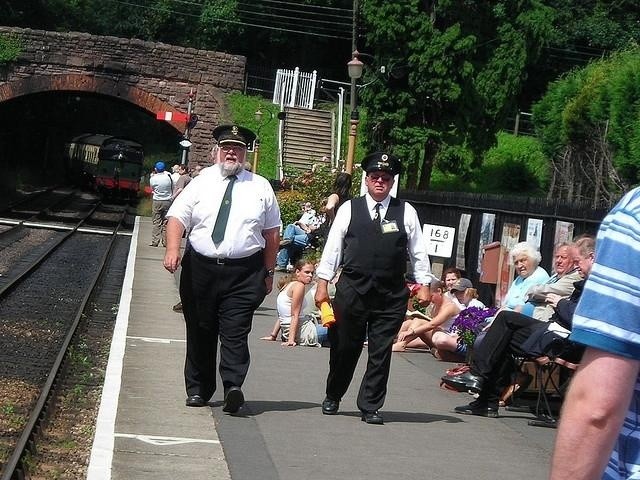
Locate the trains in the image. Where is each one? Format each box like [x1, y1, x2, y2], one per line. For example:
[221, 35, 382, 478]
[67, 132, 146, 210]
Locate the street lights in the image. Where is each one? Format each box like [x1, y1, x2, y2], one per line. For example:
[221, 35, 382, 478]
[252, 107, 274, 174]
[343, 48, 382, 176]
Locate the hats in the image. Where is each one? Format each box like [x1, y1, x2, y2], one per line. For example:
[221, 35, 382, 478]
[447, 276, 474, 294]
[211, 123, 257, 149]
[154, 161, 166, 172]
[360, 151, 408, 176]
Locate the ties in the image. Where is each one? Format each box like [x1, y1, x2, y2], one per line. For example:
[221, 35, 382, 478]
[210, 174, 238, 246]
[371, 202, 383, 228]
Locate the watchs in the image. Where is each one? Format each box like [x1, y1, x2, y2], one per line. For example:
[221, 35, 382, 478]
[266, 270, 274, 277]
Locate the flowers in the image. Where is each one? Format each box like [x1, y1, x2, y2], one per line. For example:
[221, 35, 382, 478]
[448, 305, 500, 349]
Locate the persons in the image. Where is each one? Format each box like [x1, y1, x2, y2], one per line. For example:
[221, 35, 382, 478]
[391, 267, 490, 360]
[549, 188, 640, 479]
[441, 232, 595, 418]
[260, 173, 352, 349]
[164, 125, 281, 414]
[149, 162, 202, 248]
[314, 152, 440, 425]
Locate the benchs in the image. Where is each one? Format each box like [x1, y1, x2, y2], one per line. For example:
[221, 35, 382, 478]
[512, 356, 582, 429]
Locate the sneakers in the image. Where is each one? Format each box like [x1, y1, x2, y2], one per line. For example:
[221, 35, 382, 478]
[441, 369, 485, 394]
[360, 409, 383, 427]
[322, 392, 342, 417]
[454, 398, 501, 419]
[500, 373, 535, 407]
[222, 386, 245, 416]
[186, 394, 209, 408]
